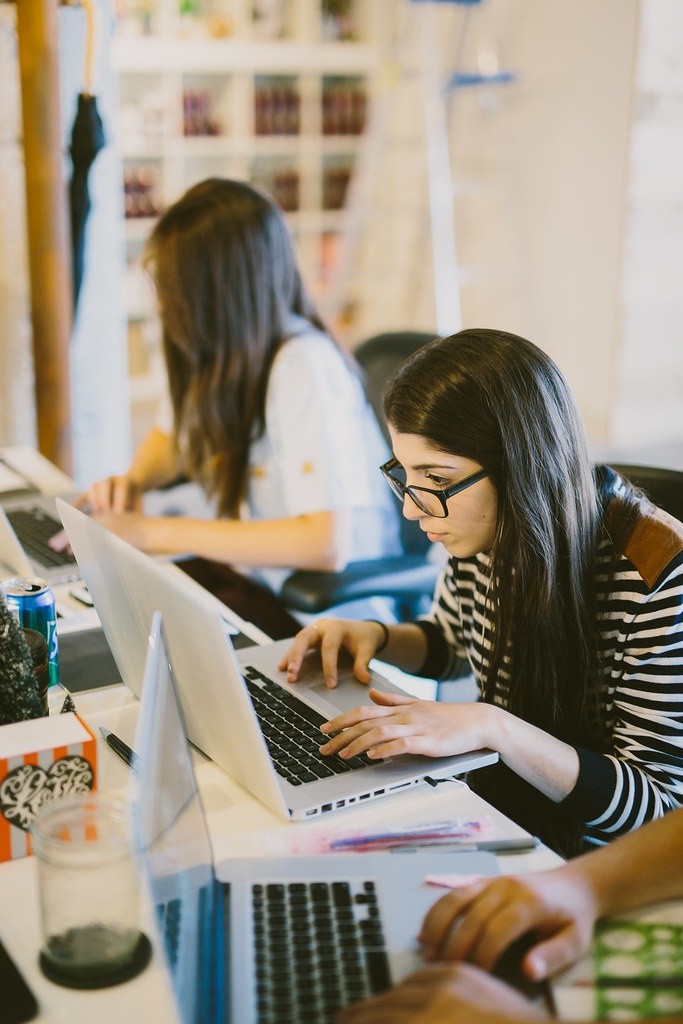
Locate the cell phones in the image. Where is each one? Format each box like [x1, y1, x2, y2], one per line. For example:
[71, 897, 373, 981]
[71, 587, 94, 606]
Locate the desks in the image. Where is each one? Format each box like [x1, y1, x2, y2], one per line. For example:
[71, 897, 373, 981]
[0, 459, 570, 1023]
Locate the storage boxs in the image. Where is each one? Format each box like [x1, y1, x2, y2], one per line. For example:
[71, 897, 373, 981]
[0, 681, 105, 866]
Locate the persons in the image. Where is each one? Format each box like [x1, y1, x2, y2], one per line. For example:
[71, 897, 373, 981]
[332, 802, 683, 1024]
[47, 169, 412, 643]
[269, 320, 683, 861]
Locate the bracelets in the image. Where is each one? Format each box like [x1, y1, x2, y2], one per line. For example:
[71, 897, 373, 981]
[363, 618, 393, 657]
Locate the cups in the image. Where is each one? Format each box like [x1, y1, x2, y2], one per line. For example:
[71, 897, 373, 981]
[26, 791, 151, 991]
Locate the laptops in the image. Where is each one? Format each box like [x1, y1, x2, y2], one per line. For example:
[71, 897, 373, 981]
[126, 609, 551, 1023]
[0, 504, 82, 586]
[54, 497, 498, 820]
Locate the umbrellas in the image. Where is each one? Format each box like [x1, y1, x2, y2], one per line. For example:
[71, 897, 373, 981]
[62, 5, 108, 329]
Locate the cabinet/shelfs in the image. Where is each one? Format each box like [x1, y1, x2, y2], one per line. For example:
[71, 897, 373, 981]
[99, 5, 387, 470]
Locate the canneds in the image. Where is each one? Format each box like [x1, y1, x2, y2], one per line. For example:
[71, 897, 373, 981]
[1, 576, 59, 688]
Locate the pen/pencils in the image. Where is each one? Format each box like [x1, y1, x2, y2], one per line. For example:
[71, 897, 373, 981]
[99, 727, 139, 771]
[391, 837, 540, 854]
[329, 831, 469, 848]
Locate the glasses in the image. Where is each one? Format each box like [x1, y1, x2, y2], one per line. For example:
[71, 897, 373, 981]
[375, 458, 492, 516]
